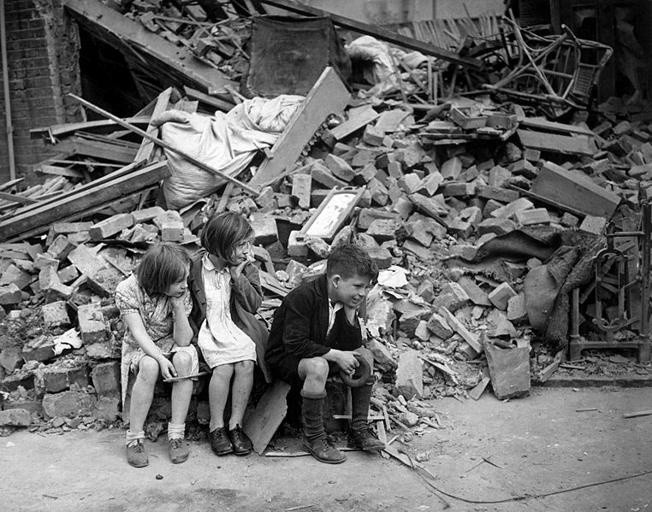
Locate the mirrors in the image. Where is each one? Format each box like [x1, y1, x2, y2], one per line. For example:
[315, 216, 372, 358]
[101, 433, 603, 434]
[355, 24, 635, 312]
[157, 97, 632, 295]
[295, 186, 366, 241]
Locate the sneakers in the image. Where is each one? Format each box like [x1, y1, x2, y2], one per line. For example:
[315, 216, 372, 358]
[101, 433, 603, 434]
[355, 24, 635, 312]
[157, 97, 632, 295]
[127, 438, 148, 468]
[303, 432, 345, 463]
[347, 426, 384, 451]
[169, 439, 189, 463]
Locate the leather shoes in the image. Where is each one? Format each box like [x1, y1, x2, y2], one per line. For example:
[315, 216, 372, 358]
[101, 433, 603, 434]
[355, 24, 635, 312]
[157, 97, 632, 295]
[229, 428, 251, 455]
[210, 427, 233, 455]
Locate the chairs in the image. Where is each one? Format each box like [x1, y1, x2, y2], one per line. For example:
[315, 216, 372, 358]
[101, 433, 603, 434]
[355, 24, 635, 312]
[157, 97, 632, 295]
[481, 15, 615, 109]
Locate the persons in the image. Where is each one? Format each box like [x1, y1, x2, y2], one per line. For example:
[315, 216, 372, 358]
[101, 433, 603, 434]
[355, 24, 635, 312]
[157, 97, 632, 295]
[188, 211, 272, 456]
[115, 243, 199, 467]
[264, 241, 386, 465]
